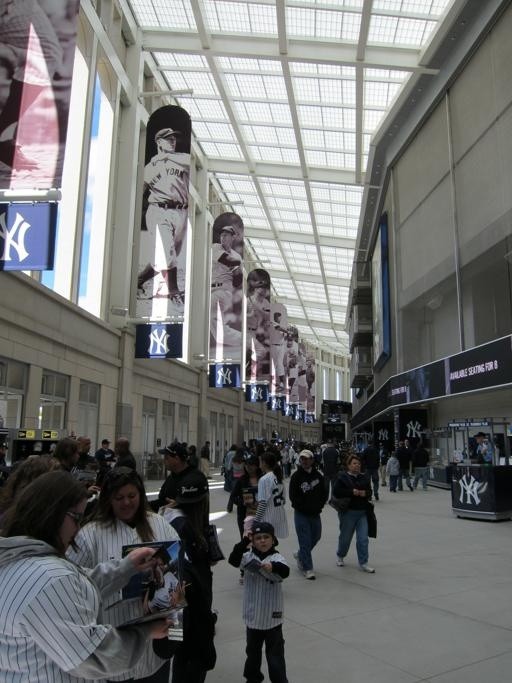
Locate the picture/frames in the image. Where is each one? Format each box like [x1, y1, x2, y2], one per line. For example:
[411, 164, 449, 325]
[369, 210, 391, 376]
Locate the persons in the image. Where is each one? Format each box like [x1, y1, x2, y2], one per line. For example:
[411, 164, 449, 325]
[77, 435, 94, 470]
[397, 439, 415, 492]
[321, 436, 353, 474]
[249, 452, 290, 539]
[227, 459, 260, 540]
[386, 451, 400, 493]
[52, 436, 82, 472]
[245, 278, 270, 383]
[212, 225, 245, 349]
[136, 128, 190, 307]
[146, 441, 209, 528]
[289, 331, 315, 403]
[113, 436, 136, 471]
[228, 521, 290, 683]
[288, 450, 330, 580]
[471, 431, 493, 465]
[331, 455, 375, 571]
[65, 467, 182, 683]
[1, 0, 73, 174]
[199, 441, 213, 480]
[0, 471, 177, 683]
[223, 433, 296, 477]
[94, 439, 114, 469]
[410, 442, 430, 491]
[380, 443, 388, 487]
[0, 453, 61, 518]
[269, 310, 288, 396]
[359, 440, 380, 501]
